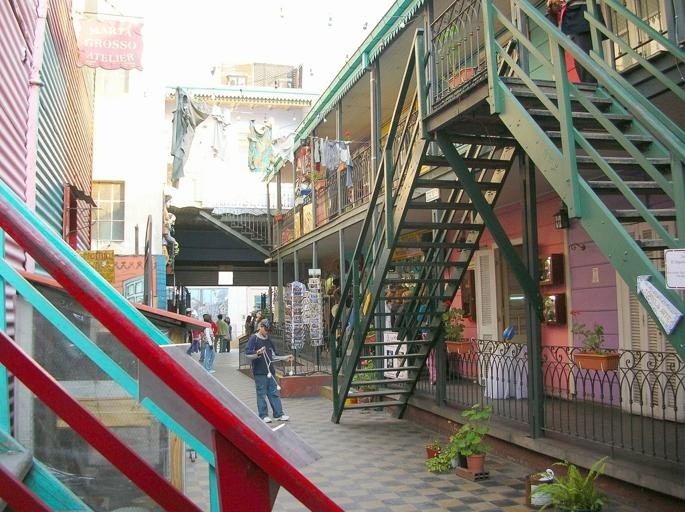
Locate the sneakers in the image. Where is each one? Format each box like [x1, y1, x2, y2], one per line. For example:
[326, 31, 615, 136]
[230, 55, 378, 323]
[273, 415, 289, 421]
[209, 370, 216, 374]
[262, 416, 272, 423]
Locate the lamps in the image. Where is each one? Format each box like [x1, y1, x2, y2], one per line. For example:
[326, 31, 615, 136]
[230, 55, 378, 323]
[552, 200, 585, 252]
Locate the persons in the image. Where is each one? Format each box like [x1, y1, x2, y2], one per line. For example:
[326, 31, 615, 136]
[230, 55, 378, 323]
[203, 315, 216, 374]
[185, 313, 233, 362]
[417, 283, 439, 385]
[244, 318, 294, 423]
[391, 290, 421, 383]
[544, 0, 614, 84]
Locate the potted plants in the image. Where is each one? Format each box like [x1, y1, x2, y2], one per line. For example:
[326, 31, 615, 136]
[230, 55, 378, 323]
[424, 401, 494, 473]
[436, 25, 477, 91]
[273, 209, 282, 221]
[309, 170, 325, 191]
[352, 358, 378, 404]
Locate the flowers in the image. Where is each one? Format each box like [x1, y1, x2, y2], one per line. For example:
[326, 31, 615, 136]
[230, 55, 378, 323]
[343, 131, 352, 141]
[440, 300, 465, 342]
[569, 310, 605, 355]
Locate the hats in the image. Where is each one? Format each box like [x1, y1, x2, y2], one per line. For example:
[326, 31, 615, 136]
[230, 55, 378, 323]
[261, 318, 271, 327]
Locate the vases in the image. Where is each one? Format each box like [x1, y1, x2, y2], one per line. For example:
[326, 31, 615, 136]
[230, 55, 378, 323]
[339, 162, 346, 172]
[444, 341, 470, 353]
[573, 351, 620, 370]
